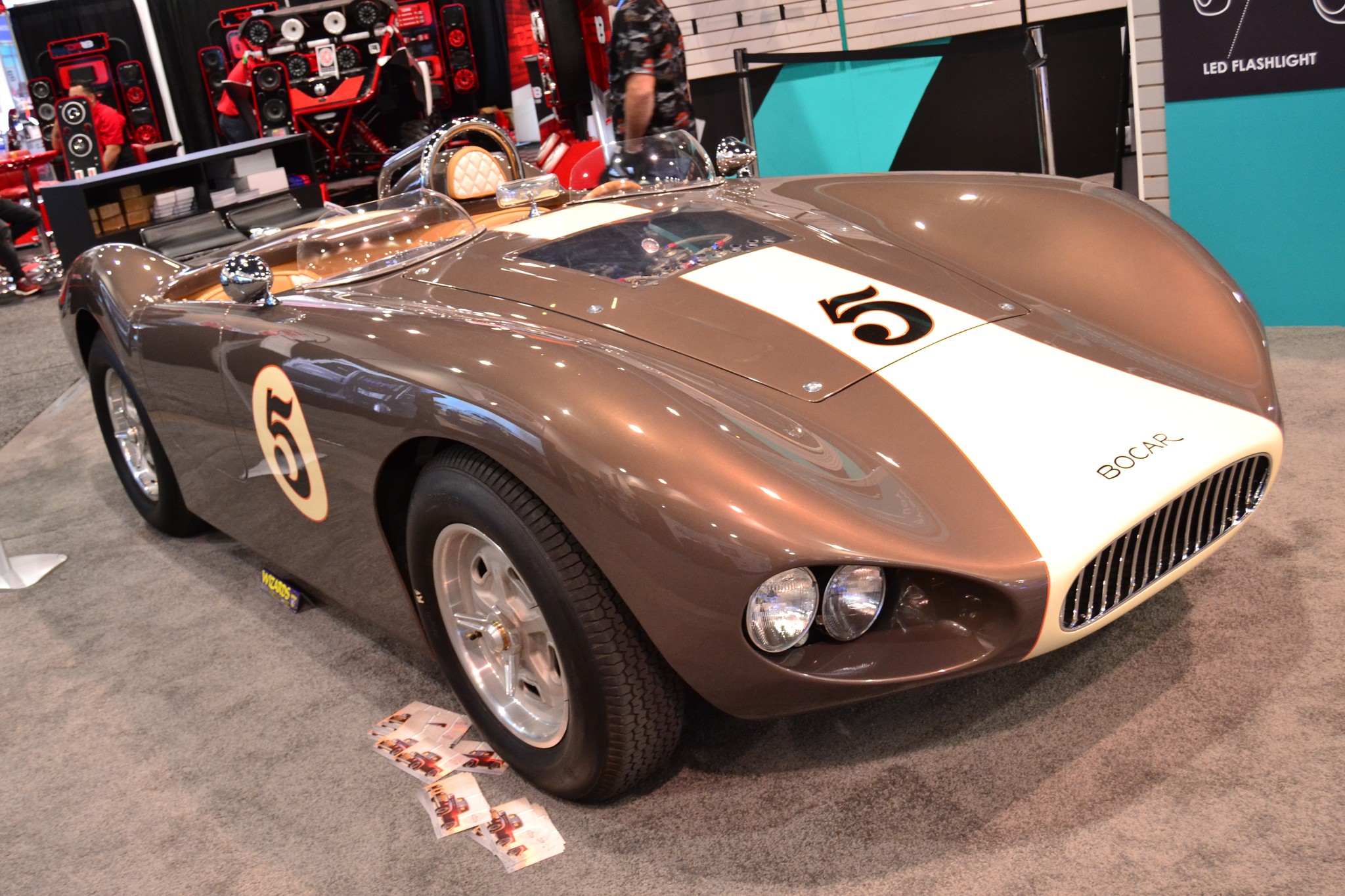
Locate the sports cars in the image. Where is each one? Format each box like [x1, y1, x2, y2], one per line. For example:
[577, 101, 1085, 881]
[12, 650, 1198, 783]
[46, 117, 1288, 809]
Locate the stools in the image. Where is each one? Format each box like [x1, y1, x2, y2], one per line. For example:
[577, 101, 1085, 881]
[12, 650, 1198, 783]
[223, 192, 338, 238]
[138, 210, 248, 260]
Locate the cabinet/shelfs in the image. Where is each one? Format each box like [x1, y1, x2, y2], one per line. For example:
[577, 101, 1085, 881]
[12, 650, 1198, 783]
[40, 132, 323, 273]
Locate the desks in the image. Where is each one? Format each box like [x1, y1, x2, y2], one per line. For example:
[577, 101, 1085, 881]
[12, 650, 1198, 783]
[0, 150, 67, 288]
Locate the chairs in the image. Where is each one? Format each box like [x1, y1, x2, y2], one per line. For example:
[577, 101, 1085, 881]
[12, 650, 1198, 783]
[130, 143, 147, 165]
[416, 114, 551, 243]
[0, 150, 59, 256]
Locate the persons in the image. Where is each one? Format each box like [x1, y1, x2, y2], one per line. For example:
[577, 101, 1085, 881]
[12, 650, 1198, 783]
[603, 0, 697, 186]
[53, 78, 125, 176]
[0, 198, 44, 295]
[216, 51, 264, 142]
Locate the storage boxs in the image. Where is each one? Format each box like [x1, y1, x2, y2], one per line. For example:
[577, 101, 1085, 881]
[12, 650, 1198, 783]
[209, 148, 288, 195]
[87, 183, 172, 234]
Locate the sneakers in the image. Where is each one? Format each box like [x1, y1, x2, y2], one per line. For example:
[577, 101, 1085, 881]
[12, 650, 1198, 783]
[15, 276, 43, 297]
